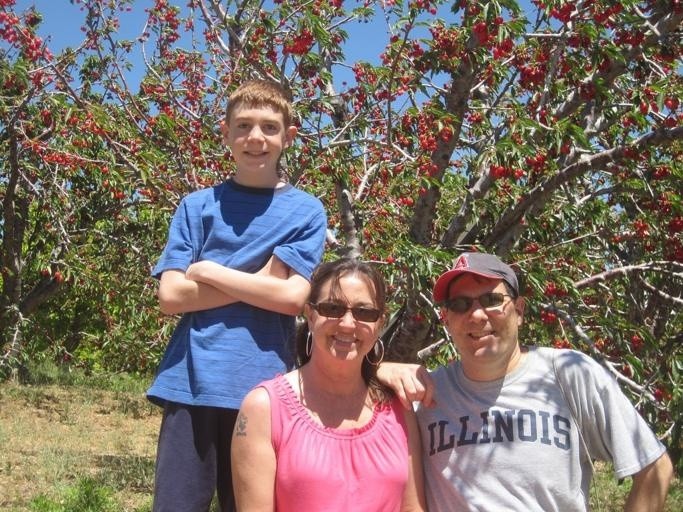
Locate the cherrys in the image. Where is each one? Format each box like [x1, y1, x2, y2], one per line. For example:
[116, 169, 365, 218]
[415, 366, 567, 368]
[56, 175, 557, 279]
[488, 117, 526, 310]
[0, 0, 683, 422]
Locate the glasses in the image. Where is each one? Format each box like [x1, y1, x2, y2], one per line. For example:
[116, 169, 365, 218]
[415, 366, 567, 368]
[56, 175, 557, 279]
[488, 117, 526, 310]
[444, 293, 515, 312]
[309, 302, 383, 322]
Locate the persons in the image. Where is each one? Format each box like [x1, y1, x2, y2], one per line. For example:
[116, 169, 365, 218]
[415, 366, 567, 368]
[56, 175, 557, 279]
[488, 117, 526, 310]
[231, 257, 427, 512]
[377, 252, 673, 512]
[148, 79, 328, 511]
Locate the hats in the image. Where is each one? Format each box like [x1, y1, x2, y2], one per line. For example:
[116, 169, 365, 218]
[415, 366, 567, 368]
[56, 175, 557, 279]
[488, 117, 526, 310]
[433, 252, 519, 302]
